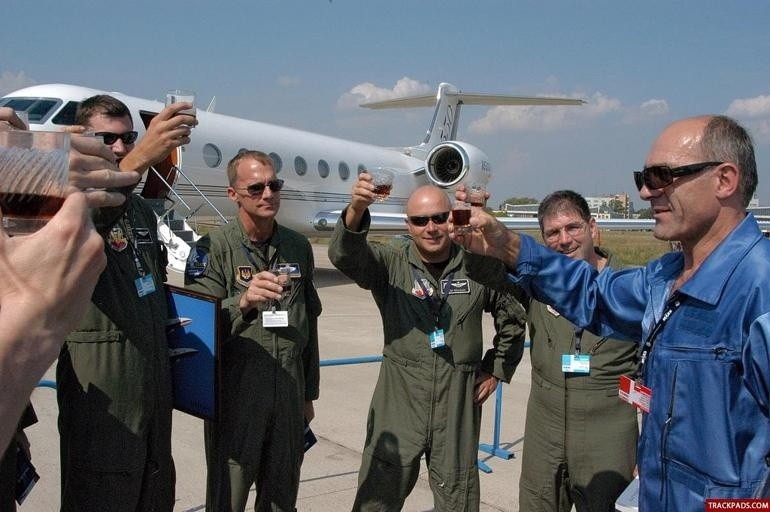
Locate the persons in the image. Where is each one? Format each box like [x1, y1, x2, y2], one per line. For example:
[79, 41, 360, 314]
[0, 134, 141, 511]
[327, 172, 526, 512]
[448, 113, 770, 512]
[0, 108, 33, 148]
[454, 185, 644, 512]
[53, 95, 199, 512]
[184, 150, 322, 512]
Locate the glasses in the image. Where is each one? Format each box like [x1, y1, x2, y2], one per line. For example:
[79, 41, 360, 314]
[407, 210, 451, 227]
[631, 159, 726, 193]
[541, 220, 587, 240]
[89, 128, 140, 147]
[233, 178, 285, 196]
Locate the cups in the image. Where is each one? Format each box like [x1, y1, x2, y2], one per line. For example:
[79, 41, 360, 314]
[363, 166, 396, 203]
[164, 90, 195, 135]
[1, 128, 71, 235]
[453, 202, 470, 235]
[465, 182, 486, 206]
[267, 266, 293, 299]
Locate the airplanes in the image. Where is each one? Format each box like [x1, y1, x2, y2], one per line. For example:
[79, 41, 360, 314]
[1, 77, 770, 246]
